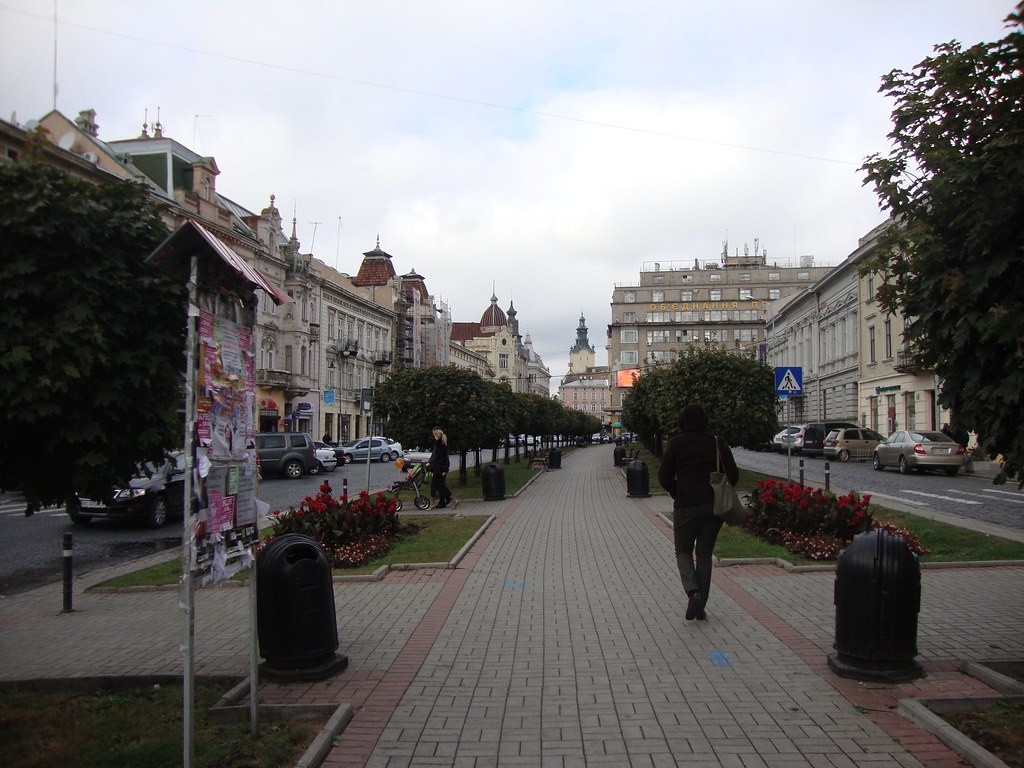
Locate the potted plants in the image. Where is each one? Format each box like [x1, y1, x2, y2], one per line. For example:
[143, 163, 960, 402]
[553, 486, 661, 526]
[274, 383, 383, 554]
[964, 446, 991, 472]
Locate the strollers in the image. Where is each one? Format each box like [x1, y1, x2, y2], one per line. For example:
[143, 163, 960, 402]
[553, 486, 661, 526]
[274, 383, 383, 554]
[387, 463, 430, 511]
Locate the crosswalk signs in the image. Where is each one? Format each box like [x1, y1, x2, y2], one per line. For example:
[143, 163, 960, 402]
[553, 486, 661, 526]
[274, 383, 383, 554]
[775, 367, 804, 399]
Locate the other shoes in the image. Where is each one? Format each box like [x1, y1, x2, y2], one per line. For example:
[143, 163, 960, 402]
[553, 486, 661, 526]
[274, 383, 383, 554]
[686, 590, 706, 620]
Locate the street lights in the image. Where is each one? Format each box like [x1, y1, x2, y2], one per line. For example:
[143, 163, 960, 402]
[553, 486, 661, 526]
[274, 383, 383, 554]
[746, 295, 776, 367]
[337, 340, 357, 446]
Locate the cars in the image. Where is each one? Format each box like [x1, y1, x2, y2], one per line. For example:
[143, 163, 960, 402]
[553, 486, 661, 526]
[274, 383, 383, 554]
[823, 427, 887, 462]
[313, 436, 404, 472]
[499, 434, 602, 448]
[773, 420, 860, 458]
[67, 448, 211, 529]
[402, 446, 433, 472]
[873, 430, 969, 475]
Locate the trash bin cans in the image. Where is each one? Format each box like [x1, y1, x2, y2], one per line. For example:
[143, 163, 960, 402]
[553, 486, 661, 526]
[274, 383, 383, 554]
[254, 531, 350, 684]
[480, 462, 506, 501]
[626, 459, 652, 498]
[548, 447, 562, 469]
[826, 526, 928, 684]
[613, 446, 626, 466]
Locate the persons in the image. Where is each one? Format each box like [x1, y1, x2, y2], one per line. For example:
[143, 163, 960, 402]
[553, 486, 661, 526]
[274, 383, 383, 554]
[940, 423, 952, 436]
[656, 402, 740, 621]
[951, 420, 969, 450]
[387, 467, 413, 493]
[425, 426, 460, 509]
[322, 430, 332, 443]
[623, 433, 629, 447]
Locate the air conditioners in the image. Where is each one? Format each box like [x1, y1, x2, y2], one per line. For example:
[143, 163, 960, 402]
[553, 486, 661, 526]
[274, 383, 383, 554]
[646, 342, 651, 346]
[642, 318, 648, 321]
[700, 317, 704, 321]
[727, 316, 731, 320]
[577, 375, 593, 380]
[615, 319, 620, 323]
[758, 316, 763, 320]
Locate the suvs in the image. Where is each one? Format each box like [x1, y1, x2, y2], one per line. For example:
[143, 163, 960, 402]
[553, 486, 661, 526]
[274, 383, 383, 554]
[254, 432, 317, 478]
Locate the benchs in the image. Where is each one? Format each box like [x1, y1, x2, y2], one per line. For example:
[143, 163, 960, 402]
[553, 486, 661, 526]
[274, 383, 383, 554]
[622, 447, 640, 466]
[538, 447, 549, 465]
[528, 449, 548, 470]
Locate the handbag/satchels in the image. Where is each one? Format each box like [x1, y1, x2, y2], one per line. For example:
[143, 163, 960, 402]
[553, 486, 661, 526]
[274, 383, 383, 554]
[710, 472, 745, 527]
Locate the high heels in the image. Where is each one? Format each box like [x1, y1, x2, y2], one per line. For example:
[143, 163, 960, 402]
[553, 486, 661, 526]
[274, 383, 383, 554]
[425, 504, 438, 511]
[453, 500, 461, 510]
